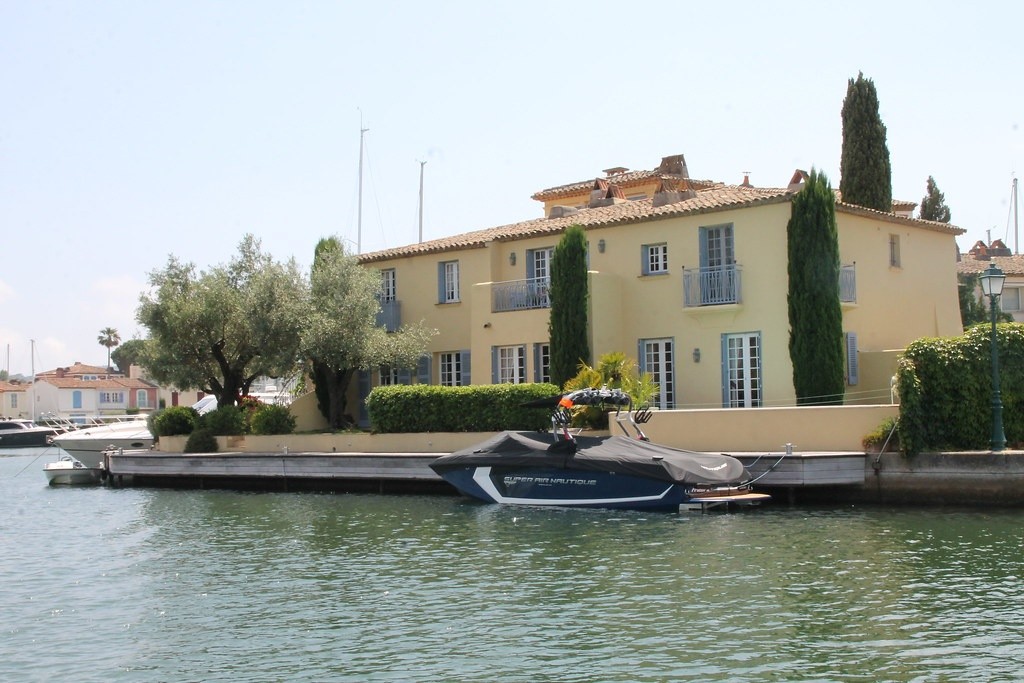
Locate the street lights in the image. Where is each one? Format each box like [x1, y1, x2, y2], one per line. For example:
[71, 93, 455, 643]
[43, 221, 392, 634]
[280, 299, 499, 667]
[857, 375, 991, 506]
[978, 260, 1007, 451]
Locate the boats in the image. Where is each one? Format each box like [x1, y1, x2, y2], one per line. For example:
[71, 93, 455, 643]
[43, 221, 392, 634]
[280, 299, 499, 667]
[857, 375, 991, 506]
[427, 387, 771, 514]
[45, 457, 103, 487]
[0, 419, 80, 446]
[52, 391, 290, 480]
